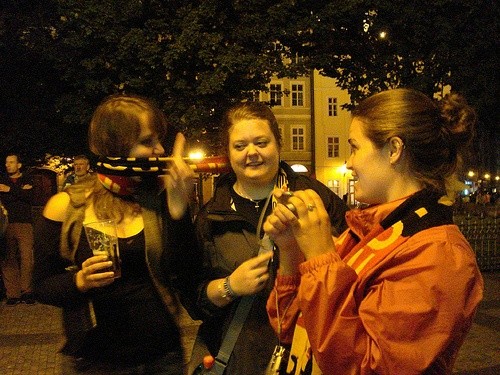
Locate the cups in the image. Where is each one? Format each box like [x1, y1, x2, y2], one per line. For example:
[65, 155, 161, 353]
[85, 219, 121, 279]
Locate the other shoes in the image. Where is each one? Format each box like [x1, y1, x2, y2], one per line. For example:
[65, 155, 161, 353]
[6, 298, 22, 306]
[25, 293, 36, 305]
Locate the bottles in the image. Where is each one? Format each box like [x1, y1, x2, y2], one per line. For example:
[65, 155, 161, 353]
[197, 355, 217, 375]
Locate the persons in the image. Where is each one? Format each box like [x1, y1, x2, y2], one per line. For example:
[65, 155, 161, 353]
[263, 88, 466, 375]
[31, 97, 207, 375]
[65, 154, 90, 187]
[187, 102, 348, 375]
[0, 153, 35, 306]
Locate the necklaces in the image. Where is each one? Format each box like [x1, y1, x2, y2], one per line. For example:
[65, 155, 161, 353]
[236, 188, 263, 213]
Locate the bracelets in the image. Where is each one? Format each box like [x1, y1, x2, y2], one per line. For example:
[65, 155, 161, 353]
[224, 276, 236, 298]
[218, 282, 230, 300]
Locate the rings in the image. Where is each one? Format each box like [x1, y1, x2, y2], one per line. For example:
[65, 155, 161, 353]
[307, 204, 315, 210]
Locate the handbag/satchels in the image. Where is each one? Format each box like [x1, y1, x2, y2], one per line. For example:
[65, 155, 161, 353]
[187, 322, 229, 375]
